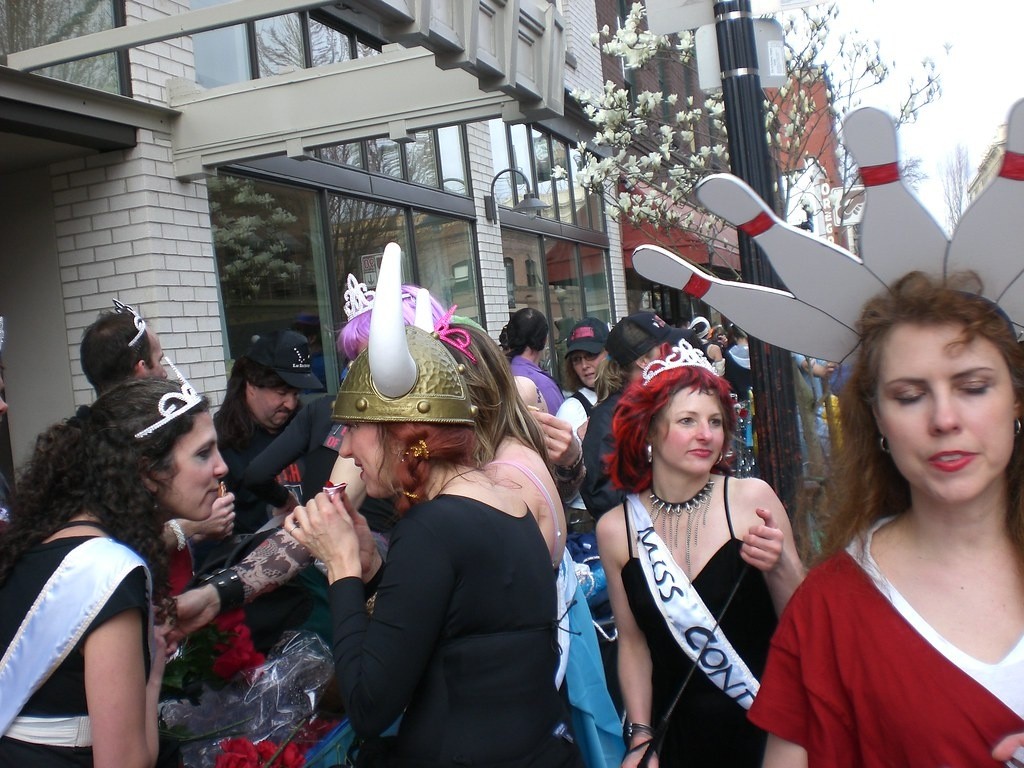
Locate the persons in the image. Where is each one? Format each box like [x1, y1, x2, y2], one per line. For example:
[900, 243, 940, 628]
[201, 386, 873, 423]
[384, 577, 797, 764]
[0, 241, 610, 767]
[594, 338, 805, 768]
[746, 270, 1024, 768]
[581, 311, 851, 526]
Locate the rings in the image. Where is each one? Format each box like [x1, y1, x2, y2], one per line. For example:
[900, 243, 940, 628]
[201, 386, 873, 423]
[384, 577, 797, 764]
[289, 526, 299, 535]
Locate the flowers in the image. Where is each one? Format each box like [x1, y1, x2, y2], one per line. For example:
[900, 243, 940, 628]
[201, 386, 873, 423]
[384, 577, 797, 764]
[165, 609, 343, 768]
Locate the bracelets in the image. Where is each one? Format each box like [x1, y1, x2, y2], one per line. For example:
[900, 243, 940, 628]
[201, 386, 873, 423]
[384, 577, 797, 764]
[627, 721, 654, 739]
[199, 567, 245, 614]
[169, 519, 186, 551]
[555, 442, 583, 483]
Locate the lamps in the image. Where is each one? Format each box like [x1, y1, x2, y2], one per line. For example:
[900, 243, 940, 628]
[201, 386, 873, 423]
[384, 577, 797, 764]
[485, 168, 551, 225]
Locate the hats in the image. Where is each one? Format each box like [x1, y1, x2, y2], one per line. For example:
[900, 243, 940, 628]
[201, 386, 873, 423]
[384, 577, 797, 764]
[605, 312, 693, 367]
[564, 317, 609, 359]
[245, 329, 326, 390]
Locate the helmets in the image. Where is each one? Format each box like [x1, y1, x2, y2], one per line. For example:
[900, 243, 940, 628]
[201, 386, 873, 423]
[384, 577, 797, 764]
[330, 241, 479, 429]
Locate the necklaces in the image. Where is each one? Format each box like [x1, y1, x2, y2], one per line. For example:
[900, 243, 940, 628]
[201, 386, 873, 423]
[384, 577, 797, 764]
[438, 469, 478, 495]
[649, 479, 715, 575]
